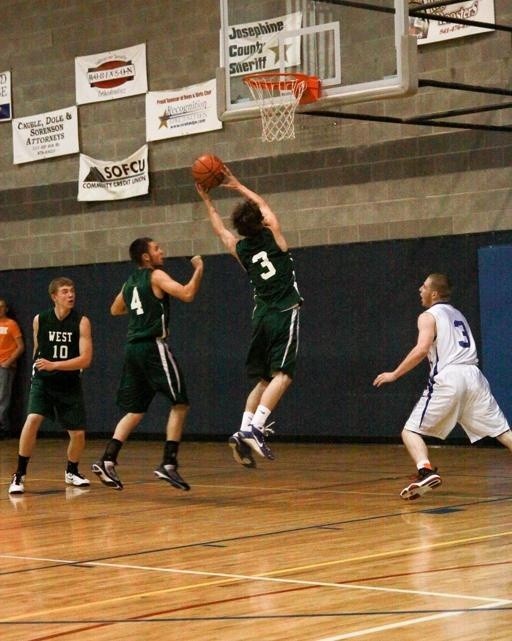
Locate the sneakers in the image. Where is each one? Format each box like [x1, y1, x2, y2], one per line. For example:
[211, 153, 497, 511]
[153, 462, 191, 492]
[9, 472, 26, 494]
[399, 466, 443, 502]
[229, 431, 257, 470]
[91, 456, 123, 491]
[241, 424, 276, 461]
[65, 469, 91, 487]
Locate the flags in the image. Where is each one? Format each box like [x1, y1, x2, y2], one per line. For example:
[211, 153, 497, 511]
[143, 76, 225, 144]
[73, 41, 149, 108]
[12, 104, 81, 169]
[218, 10, 303, 79]
[76, 140, 151, 204]
[1, 69, 15, 122]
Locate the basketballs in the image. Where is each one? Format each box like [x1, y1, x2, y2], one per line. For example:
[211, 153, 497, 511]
[192, 155, 225, 190]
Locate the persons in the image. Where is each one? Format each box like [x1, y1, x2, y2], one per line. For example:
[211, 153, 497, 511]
[398, 504, 510, 641]
[193, 163, 304, 471]
[1, 298, 26, 442]
[95, 481, 187, 594]
[372, 273, 510, 503]
[7, 481, 92, 595]
[8, 276, 95, 495]
[89, 236, 204, 493]
